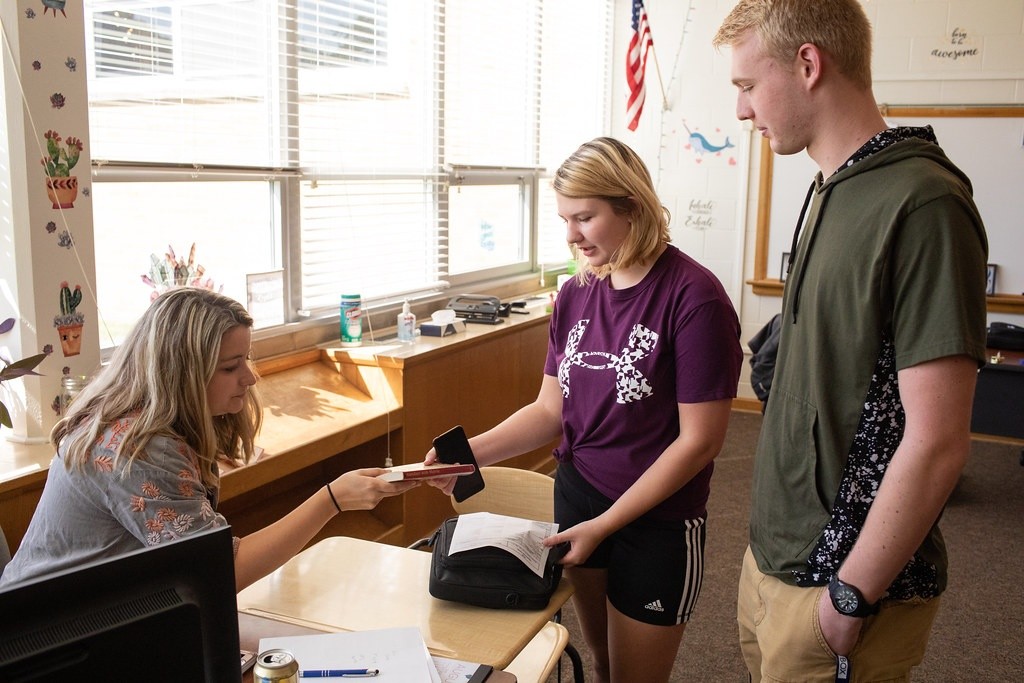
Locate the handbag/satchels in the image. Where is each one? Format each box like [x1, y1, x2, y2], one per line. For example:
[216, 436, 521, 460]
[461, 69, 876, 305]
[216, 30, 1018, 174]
[972, 362, 1024, 438]
[430, 515, 571, 610]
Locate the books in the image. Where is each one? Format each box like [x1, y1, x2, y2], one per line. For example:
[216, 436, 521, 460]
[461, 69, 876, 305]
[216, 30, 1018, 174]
[376, 459, 476, 482]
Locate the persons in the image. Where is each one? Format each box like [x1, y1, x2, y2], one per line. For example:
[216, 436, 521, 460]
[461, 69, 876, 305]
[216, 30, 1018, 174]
[0, 287, 423, 595]
[713, 0, 989, 683]
[425, 136, 744, 683]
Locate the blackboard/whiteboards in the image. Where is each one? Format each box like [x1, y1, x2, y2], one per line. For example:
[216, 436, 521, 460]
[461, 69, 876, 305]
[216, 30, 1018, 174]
[744, 102, 1023, 315]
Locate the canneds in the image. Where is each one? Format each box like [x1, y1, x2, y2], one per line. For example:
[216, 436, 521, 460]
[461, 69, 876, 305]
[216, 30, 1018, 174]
[253, 648, 300, 683]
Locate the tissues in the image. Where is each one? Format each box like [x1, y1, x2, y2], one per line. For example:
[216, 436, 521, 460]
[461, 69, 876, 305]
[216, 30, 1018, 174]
[419, 309, 467, 338]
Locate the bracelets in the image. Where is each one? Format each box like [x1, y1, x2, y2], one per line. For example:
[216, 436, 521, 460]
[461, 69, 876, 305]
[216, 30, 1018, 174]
[326, 482, 344, 515]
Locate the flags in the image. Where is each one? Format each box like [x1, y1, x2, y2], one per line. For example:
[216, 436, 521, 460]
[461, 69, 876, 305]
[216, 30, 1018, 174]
[624, 0, 654, 131]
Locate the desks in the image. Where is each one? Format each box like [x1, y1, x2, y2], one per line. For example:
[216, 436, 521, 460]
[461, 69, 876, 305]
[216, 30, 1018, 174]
[321, 287, 565, 547]
[221, 536, 576, 683]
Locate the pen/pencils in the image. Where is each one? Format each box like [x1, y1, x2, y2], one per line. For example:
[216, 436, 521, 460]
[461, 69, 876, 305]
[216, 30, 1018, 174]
[297, 668, 379, 678]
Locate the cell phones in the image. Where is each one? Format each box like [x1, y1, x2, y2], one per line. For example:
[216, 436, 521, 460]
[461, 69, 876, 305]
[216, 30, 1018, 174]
[433, 425, 485, 503]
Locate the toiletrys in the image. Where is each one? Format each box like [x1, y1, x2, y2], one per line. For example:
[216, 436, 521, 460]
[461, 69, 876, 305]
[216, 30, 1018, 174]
[339, 294, 363, 348]
[396, 296, 417, 343]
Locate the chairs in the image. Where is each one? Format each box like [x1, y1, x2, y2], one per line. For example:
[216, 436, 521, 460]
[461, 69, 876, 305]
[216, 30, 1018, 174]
[410, 466, 586, 683]
[971, 365, 1024, 446]
[986, 322, 1024, 353]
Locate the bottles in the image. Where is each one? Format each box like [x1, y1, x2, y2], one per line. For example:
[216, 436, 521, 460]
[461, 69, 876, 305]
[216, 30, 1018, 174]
[397, 297, 416, 344]
[59, 375, 90, 419]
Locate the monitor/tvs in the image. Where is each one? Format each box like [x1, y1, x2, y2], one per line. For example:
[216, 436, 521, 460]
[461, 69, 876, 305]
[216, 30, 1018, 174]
[0, 525, 241, 683]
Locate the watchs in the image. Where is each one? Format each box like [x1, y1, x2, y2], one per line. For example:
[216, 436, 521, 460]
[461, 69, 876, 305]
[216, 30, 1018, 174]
[827, 574, 881, 619]
